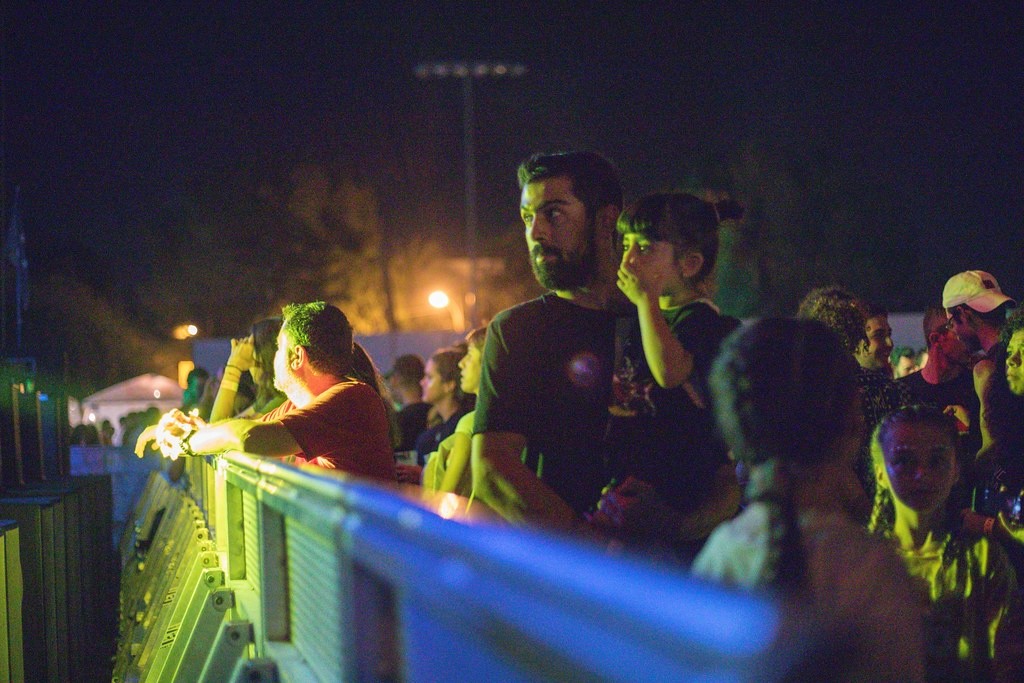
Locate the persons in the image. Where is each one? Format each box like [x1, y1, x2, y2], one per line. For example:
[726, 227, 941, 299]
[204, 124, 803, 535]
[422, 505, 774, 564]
[79, 150, 1024, 683]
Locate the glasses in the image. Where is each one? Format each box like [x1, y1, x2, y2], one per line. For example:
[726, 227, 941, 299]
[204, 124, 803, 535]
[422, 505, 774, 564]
[943, 311, 961, 330]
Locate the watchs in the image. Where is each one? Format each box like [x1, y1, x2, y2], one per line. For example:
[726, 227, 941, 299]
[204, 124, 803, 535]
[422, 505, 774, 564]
[179, 430, 200, 457]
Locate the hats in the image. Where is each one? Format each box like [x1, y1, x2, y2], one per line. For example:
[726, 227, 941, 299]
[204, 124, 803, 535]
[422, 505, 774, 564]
[942, 271, 1017, 314]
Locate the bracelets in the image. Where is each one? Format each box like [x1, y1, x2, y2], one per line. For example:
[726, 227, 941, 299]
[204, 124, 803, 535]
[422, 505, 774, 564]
[983, 516, 996, 535]
